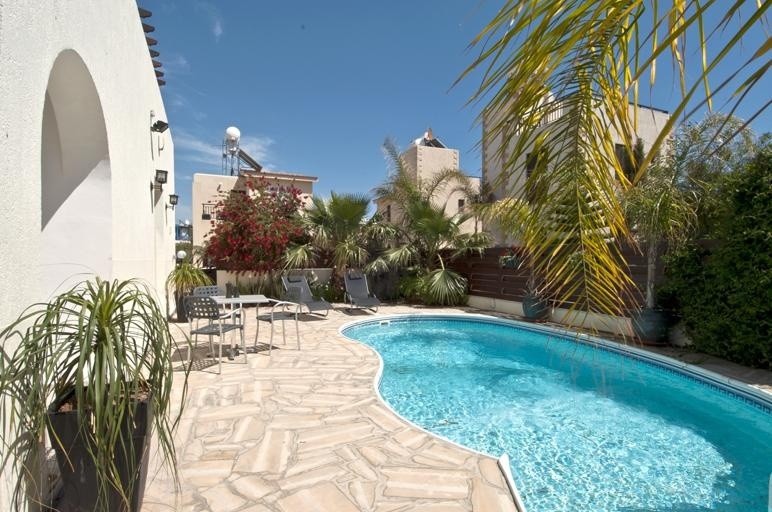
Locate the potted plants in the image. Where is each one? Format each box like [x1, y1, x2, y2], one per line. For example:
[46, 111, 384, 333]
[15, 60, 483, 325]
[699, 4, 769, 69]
[617, 179, 701, 343]
[0, 269, 168, 512]
[468, 198, 559, 320]
[165, 248, 213, 322]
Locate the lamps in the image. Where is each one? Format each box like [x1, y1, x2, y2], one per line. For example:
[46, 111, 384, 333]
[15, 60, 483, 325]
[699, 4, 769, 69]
[164, 194, 178, 211]
[150, 120, 168, 133]
[151, 169, 168, 192]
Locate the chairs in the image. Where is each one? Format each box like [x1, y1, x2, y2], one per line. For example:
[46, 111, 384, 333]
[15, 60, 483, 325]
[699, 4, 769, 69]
[174, 267, 383, 375]
